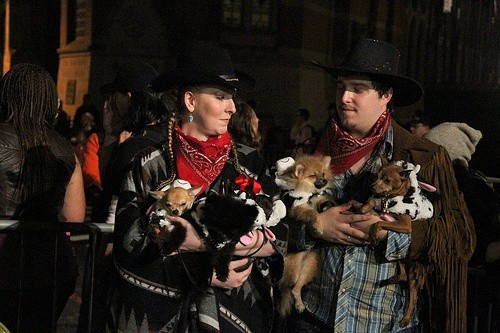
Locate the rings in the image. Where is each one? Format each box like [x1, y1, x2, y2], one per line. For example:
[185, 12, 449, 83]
[346, 236, 350, 242]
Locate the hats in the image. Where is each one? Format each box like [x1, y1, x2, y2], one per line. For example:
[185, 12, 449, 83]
[175, 57, 240, 95]
[408, 109, 435, 125]
[311, 34, 423, 107]
[424, 122, 483, 168]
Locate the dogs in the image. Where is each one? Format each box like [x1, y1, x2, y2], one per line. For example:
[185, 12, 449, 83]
[147, 185, 277, 223]
[273, 152, 334, 315]
[360, 151, 435, 327]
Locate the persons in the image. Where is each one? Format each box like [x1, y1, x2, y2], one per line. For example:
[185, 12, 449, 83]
[76, 44, 286, 333]
[0, 63, 85, 333]
[274, 36, 477, 333]
[53, 81, 500, 333]
